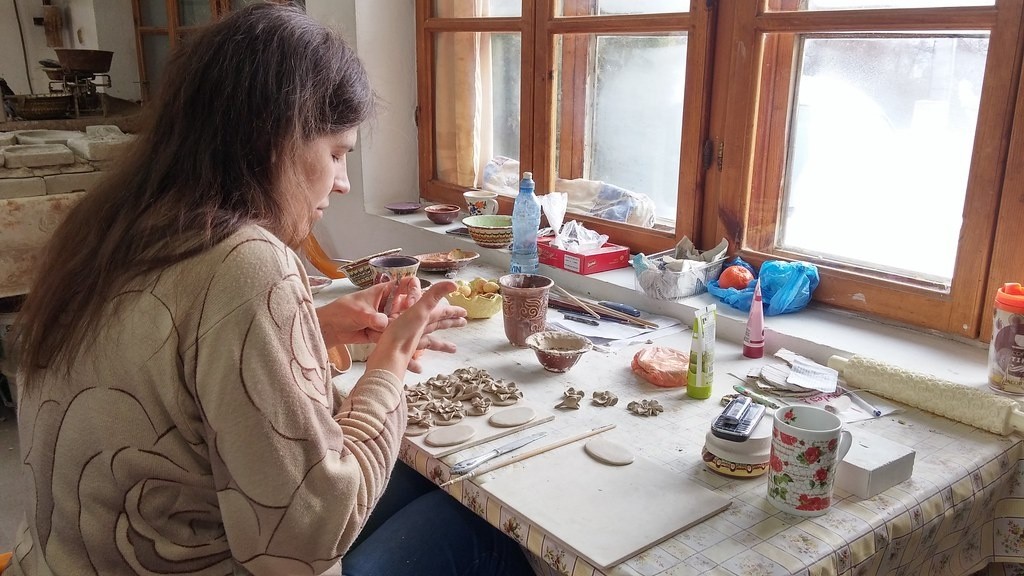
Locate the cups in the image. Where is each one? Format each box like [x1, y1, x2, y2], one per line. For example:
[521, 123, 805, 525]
[766, 405, 852, 517]
[499, 273, 554, 349]
[368, 255, 420, 313]
[463, 191, 499, 216]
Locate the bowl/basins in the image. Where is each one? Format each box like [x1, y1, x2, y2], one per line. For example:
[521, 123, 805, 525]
[461, 215, 514, 249]
[336, 248, 403, 290]
[424, 204, 460, 224]
[525, 331, 594, 373]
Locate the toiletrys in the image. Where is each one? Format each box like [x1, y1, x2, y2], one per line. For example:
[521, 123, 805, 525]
[687, 303, 717, 399]
[742, 277, 765, 358]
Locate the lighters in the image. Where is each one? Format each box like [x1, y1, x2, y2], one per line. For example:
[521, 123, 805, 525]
[726, 393, 751, 426]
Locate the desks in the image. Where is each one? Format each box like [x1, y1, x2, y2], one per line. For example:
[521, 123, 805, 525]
[310, 261, 1023, 576]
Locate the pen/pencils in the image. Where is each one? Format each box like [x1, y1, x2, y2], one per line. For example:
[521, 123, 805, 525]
[844, 389, 881, 417]
[733, 383, 779, 409]
[564, 315, 600, 326]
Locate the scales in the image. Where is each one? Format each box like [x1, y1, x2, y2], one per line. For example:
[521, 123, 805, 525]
[39, 47, 114, 117]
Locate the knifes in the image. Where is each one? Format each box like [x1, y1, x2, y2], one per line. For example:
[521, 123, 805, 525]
[575, 295, 640, 316]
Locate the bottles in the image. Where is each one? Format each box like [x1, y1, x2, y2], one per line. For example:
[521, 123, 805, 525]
[509, 171, 541, 274]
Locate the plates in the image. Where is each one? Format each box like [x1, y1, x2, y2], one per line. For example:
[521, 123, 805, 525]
[411, 251, 480, 272]
[383, 201, 421, 214]
[307, 275, 332, 295]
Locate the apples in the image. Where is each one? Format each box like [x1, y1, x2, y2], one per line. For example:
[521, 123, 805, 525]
[719, 265, 754, 290]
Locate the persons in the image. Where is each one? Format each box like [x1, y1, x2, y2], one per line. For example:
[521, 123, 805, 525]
[0, 0, 472, 576]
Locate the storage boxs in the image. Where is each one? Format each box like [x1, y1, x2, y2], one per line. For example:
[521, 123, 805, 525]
[836, 422, 916, 501]
[538, 234, 630, 275]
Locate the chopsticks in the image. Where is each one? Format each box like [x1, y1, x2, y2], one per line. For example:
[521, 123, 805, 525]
[548, 284, 659, 330]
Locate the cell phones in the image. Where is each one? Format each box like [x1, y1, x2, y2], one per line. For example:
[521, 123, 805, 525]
[711, 399, 765, 442]
